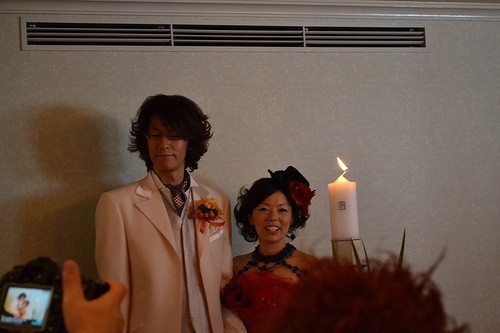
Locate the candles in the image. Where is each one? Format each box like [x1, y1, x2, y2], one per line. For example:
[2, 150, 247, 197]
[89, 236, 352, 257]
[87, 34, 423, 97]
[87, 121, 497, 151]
[327, 156, 359, 240]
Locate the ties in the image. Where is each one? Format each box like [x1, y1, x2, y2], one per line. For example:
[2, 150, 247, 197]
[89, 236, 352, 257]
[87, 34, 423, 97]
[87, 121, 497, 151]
[164, 180, 188, 218]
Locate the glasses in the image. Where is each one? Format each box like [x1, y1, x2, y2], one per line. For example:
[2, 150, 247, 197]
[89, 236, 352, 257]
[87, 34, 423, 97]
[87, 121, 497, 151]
[145, 129, 183, 142]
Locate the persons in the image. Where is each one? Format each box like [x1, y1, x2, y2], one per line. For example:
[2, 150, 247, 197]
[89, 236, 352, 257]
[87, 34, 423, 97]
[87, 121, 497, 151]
[94, 94, 248, 333]
[60, 258, 126, 332]
[219, 165, 324, 333]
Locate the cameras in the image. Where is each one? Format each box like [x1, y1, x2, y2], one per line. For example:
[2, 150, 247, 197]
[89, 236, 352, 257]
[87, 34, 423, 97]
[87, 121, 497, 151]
[1, 257, 110, 333]
